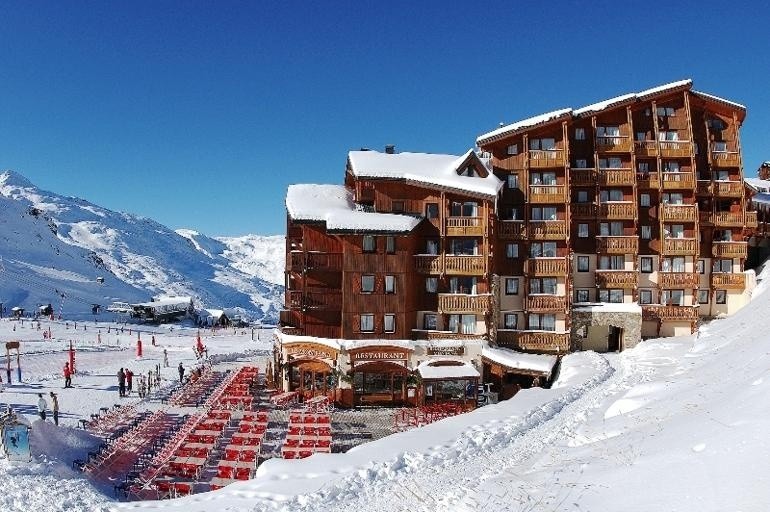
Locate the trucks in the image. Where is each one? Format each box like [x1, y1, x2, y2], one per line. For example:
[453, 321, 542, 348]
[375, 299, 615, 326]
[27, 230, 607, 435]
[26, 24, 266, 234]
[105, 302, 134, 314]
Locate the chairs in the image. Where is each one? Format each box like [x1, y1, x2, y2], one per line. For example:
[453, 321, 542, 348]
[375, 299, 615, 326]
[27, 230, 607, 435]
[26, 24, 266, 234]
[73, 367, 330, 502]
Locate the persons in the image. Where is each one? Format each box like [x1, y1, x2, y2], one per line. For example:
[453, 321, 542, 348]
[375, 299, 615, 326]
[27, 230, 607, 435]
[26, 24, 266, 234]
[164, 349, 169, 368]
[63, 362, 72, 388]
[138, 372, 145, 398]
[189, 370, 198, 384]
[37, 393, 47, 421]
[125, 368, 134, 390]
[117, 367, 126, 397]
[178, 362, 185, 382]
[49, 391, 59, 426]
